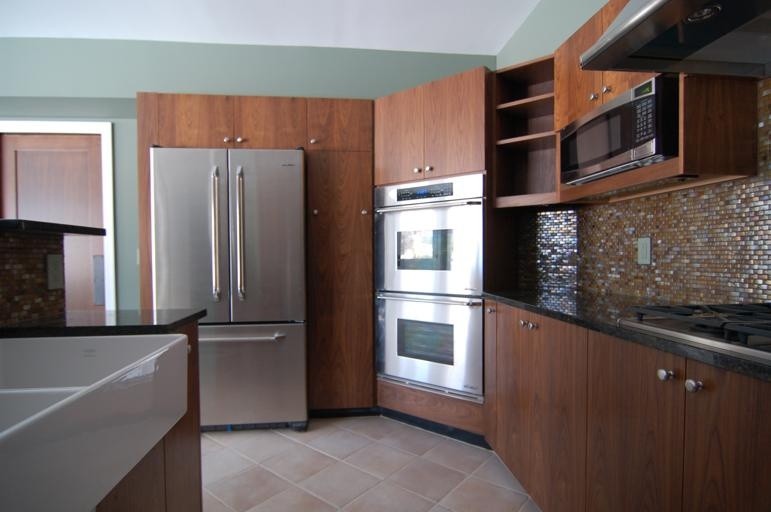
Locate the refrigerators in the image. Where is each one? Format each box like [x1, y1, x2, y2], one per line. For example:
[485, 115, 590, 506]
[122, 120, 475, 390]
[150, 147, 307, 430]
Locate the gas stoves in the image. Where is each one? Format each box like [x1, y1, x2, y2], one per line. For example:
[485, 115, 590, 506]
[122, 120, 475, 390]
[618, 302, 771, 362]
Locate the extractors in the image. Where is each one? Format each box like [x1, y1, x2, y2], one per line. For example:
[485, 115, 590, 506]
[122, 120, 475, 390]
[578, 0, 771, 77]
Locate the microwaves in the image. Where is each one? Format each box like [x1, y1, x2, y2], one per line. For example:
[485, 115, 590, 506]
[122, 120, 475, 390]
[560, 71, 681, 187]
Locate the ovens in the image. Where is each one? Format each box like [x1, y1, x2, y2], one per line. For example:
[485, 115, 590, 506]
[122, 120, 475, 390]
[375, 172, 486, 406]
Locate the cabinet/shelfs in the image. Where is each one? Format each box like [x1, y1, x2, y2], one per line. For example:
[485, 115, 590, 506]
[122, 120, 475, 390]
[373, 66, 492, 185]
[135, 91, 378, 149]
[305, 150, 374, 416]
